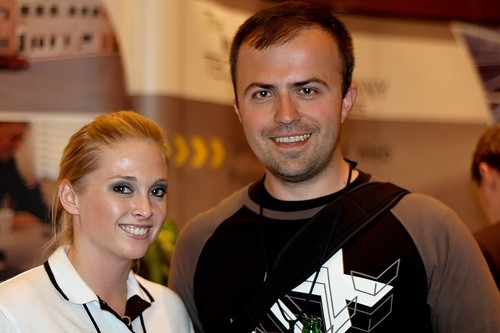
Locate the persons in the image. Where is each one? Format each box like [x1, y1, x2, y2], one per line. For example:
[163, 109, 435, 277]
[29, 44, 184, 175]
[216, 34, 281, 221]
[470, 120, 500, 293]
[1, 109, 195, 333]
[169, 2, 499, 333]
[0, 120, 51, 221]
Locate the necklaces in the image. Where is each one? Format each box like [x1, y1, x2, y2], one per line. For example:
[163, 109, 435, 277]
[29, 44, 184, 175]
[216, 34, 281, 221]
[257, 160, 353, 332]
